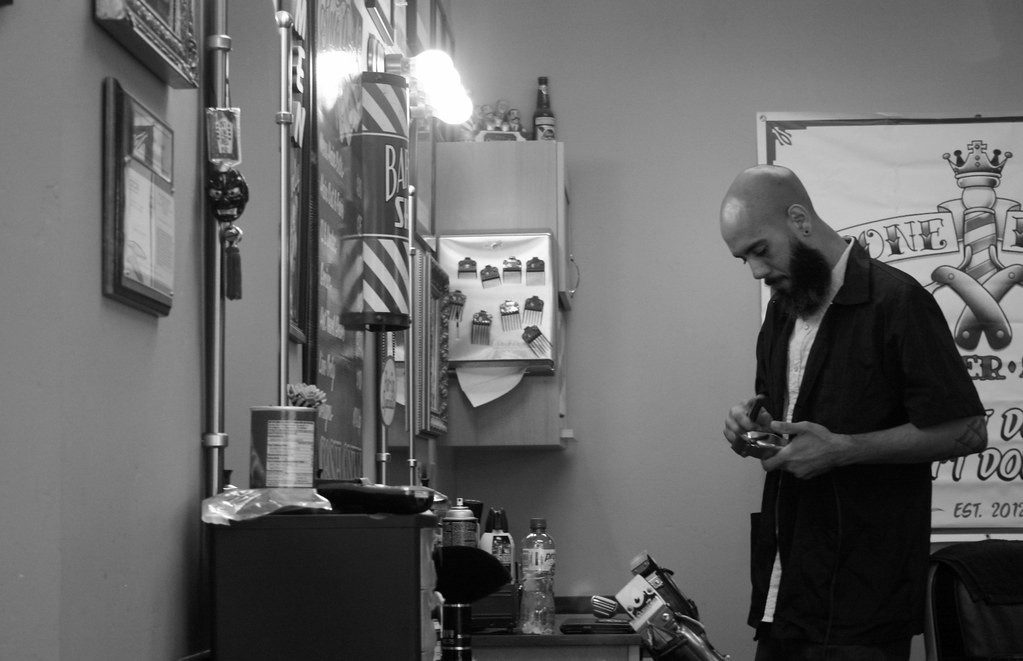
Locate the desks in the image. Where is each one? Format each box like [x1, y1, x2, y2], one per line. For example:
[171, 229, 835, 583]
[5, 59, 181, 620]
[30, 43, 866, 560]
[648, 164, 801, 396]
[471, 596, 642, 661]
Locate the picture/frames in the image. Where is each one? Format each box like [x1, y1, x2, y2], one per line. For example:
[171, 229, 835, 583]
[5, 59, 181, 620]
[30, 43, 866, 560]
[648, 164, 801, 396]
[365, 0, 456, 58]
[93, 0, 202, 90]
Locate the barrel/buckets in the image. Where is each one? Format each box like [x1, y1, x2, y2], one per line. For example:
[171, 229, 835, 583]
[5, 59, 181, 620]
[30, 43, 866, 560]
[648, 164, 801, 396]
[249, 405, 318, 489]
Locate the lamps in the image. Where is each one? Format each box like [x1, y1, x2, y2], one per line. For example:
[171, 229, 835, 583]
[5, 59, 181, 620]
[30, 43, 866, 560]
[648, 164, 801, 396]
[359, 71, 415, 332]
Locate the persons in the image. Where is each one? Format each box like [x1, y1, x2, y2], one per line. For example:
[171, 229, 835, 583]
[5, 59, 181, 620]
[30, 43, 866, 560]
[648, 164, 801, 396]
[720, 163, 989, 660]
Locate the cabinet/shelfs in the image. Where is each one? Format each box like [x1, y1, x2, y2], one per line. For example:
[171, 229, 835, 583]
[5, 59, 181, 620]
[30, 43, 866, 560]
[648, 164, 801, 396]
[433, 140, 578, 450]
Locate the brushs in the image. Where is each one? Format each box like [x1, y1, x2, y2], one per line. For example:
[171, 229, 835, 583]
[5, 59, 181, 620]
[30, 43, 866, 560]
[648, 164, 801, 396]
[432, 545, 511, 661]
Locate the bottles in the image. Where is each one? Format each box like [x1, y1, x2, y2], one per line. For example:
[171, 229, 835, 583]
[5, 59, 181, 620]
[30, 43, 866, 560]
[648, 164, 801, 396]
[442, 498, 478, 547]
[480, 506, 516, 585]
[519, 518, 557, 635]
[533, 77, 555, 140]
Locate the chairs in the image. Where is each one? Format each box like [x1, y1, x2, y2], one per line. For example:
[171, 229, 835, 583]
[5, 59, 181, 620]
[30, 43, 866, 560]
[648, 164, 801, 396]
[924, 539, 1023, 660]
